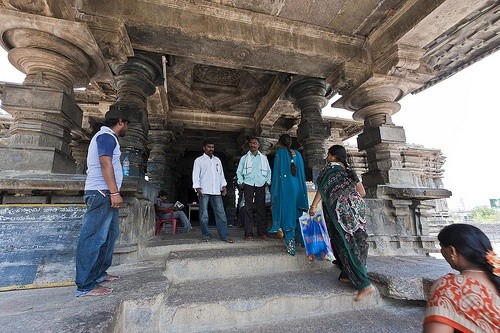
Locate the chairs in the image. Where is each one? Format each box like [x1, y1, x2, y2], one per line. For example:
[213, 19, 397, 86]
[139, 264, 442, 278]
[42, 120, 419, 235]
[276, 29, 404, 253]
[156, 206, 179, 235]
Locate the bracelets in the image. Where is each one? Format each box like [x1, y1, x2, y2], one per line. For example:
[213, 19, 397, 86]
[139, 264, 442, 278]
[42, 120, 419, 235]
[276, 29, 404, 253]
[310, 205, 317, 208]
[110, 191, 120, 196]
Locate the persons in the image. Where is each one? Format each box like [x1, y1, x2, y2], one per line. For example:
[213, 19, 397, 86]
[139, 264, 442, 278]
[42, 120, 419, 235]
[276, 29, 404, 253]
[308, 145, 374, 302]
[73, 110, 132, 297]
[154, 189, 198, 233]
[236, 138, 273, 243]
[192, 139, 235, 243]
[269, 134, 309, 257]
[422, 223, 500, 333]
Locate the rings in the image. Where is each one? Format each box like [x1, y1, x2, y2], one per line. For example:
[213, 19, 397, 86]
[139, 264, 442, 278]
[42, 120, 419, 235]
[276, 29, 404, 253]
[114, 205, 120, 208]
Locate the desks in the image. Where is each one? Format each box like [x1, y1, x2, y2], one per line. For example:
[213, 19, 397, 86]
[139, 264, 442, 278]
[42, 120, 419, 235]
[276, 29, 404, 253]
[188, 203, 199, 223]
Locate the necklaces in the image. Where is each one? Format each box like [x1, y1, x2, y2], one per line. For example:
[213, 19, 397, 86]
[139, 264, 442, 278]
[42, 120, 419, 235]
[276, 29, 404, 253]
[463, 269, 484, 274]
